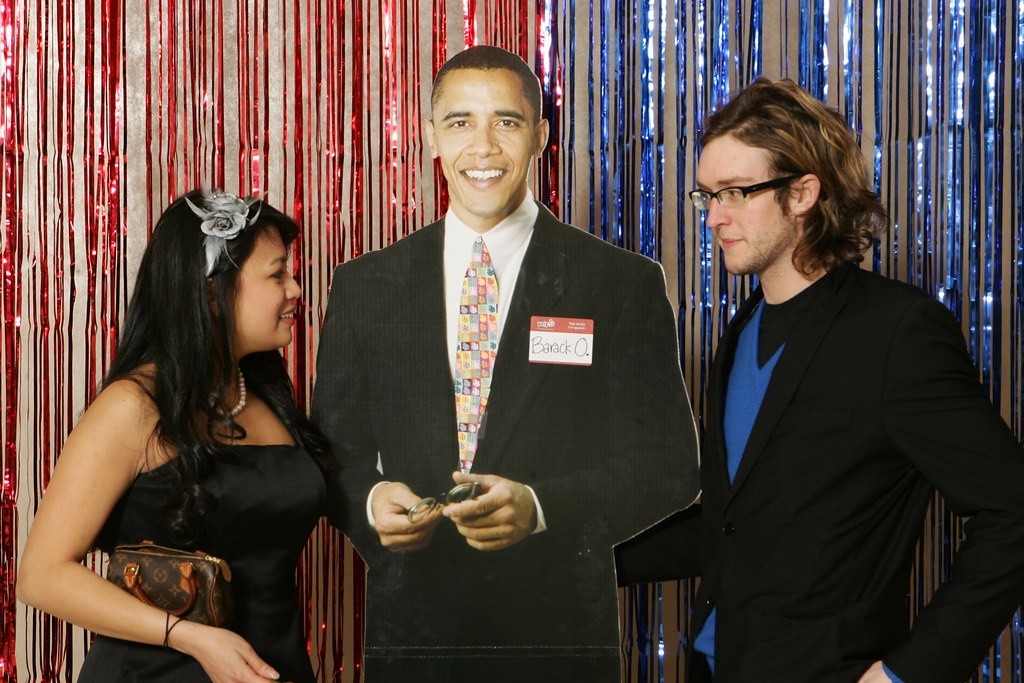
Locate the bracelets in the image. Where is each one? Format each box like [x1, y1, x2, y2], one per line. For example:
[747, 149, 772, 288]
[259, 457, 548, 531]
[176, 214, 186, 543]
[160, 610, 184, 653]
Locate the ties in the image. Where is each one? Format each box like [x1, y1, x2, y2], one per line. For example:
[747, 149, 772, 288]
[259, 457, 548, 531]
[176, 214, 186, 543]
[454, 235, 498, 474]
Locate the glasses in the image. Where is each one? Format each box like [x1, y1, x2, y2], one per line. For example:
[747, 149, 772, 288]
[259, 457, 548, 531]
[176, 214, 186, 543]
[689, 175, 829, 209]
[408, 482, 484, 523]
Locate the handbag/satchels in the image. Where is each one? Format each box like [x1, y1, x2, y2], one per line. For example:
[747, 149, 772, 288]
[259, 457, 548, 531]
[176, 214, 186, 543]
[105, 538, 235, 630]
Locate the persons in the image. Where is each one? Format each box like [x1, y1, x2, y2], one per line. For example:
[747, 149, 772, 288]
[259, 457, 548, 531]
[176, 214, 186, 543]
[16, 191, 341, 682]
[618, 75, 1024, 683]
[315, 45, 707, 683]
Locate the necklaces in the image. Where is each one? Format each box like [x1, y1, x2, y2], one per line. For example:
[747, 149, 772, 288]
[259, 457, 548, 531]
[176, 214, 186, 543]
[195, 367, 248, 416]
